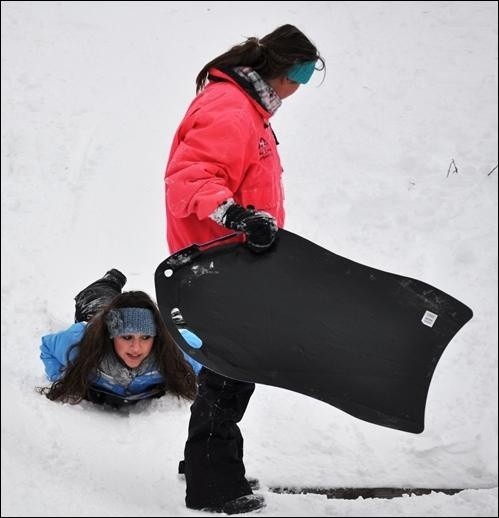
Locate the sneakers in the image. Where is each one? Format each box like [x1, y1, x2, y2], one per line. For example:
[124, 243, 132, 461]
[188, 495, 264, 515]
[103, 268, 127, 290]
[245, 475, 260, 490]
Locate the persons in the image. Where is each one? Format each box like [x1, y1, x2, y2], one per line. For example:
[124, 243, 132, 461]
[35, 264, 206, 410]
[159, 22, 323, 518]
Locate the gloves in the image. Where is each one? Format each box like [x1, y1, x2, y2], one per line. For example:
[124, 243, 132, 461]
[210, 199, 278, 253]
